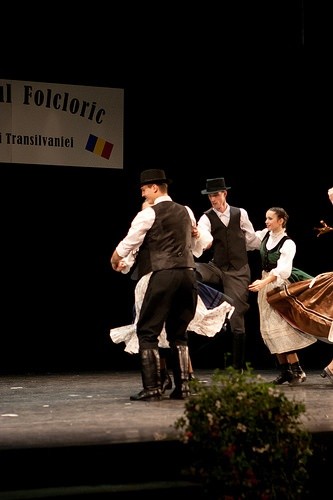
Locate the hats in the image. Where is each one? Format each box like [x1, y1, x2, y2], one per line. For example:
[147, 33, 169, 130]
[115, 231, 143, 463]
[137, 169, 172, 185]
[201, 178, 231, 194]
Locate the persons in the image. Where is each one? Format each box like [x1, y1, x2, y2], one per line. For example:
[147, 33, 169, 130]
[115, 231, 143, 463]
[188, 176, 266, 379]
[313, 187, 333, 385]
[243, 205, 315, 387]
[113, 189, 199, 395]
[111, 171, 199, 402]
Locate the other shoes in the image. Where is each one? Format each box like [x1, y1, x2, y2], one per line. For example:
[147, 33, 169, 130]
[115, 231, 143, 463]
[270, 371, 293, 385]
[288, 372, 306, 387]
[324, 360, 333, 381]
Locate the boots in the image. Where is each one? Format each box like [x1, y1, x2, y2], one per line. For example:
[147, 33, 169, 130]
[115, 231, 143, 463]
[129, 347, 162, 401]
[162, 344, 190, 400]
[232, 333, 249, 376]
[157, 348, 173, 394]
